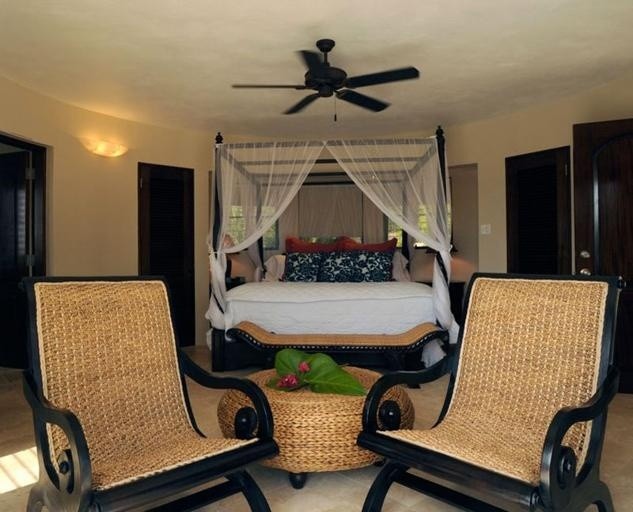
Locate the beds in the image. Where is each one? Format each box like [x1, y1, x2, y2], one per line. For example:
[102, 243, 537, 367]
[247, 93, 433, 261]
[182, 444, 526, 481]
[208, 125, 460, 372]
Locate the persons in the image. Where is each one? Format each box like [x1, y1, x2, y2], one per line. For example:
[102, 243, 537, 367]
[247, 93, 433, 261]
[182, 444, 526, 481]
[221, 233, 235, 249]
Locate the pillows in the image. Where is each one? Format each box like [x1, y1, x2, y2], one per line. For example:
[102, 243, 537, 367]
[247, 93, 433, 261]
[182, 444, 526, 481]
[285, 238, 335, 252]
[319, 251, 363, 282]
[280, 252, 323, 282]
[336, 236, 398, 253]
[350, 250, 394, 281]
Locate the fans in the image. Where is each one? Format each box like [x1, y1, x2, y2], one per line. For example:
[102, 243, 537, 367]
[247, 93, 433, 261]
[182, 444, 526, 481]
[231, 39, 420, 122]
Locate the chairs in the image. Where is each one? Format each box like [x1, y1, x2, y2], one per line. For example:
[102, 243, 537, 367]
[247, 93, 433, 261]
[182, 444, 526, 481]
[22, 275, 279, 512]
[357, 271, 629, 512]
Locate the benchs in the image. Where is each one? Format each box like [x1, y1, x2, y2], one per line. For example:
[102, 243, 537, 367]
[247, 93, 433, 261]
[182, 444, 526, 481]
[226, 320, 448, 389]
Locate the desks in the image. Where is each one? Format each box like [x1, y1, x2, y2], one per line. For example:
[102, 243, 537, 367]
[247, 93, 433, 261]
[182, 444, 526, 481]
[218, 363, 414, 490]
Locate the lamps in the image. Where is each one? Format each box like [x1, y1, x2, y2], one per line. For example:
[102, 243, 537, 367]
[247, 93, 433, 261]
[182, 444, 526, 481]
[424, 239, 458, 255]
[218, 234, 240, 283]
[80, 137, 128, 157]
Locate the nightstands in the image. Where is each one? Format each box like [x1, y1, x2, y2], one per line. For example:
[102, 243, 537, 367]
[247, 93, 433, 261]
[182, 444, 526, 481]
[225, 276, 245, 291]
[415, 280, 464, 328]
